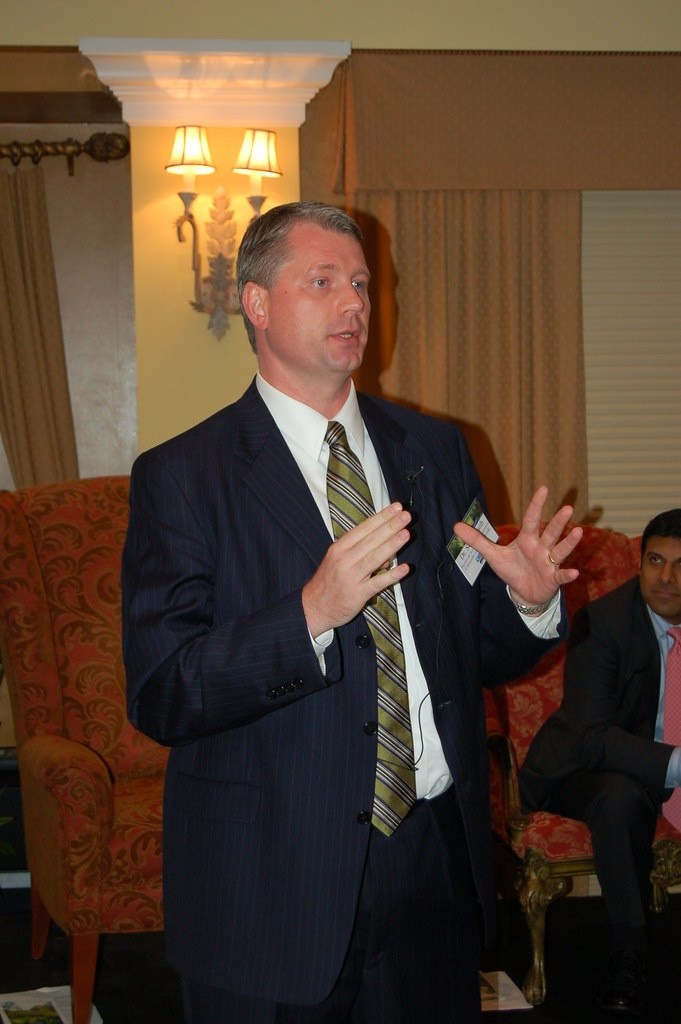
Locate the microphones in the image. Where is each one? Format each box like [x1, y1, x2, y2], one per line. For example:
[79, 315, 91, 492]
[408, 465, 426, 483]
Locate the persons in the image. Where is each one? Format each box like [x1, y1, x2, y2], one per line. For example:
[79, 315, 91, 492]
[518, 509, 681, 1015]
[121, 200, 583, 1024]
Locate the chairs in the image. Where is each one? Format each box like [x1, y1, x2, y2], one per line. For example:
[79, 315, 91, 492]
[0, 473, 680, 1024]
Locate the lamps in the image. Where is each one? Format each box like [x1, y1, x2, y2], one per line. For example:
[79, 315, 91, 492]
[224, 128, 283, 314]
[166, 124, 219, 314]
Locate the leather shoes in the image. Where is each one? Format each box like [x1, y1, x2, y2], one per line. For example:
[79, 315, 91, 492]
[598, 952, 645, 1008]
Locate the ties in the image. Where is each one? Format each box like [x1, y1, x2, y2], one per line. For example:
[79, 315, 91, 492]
[323, 420, 418, 836]
[662, 627, 681, 831]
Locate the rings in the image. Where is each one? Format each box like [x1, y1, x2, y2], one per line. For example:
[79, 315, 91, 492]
[547, 549, 560, 565]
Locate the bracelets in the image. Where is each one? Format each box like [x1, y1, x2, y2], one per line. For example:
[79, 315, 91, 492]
[505, 584, 559, 614]
